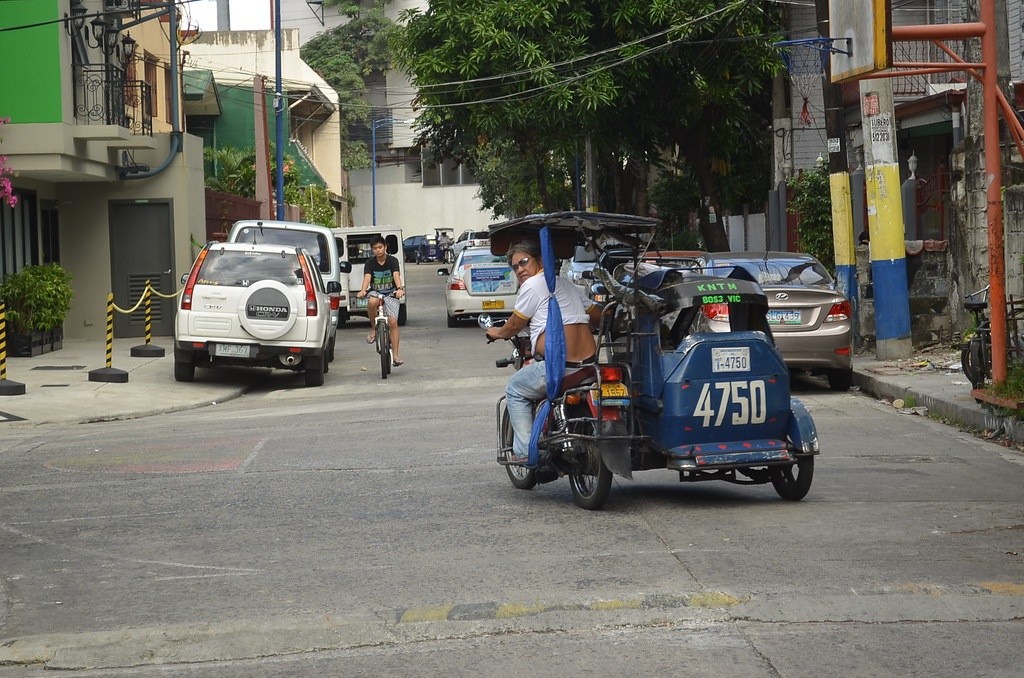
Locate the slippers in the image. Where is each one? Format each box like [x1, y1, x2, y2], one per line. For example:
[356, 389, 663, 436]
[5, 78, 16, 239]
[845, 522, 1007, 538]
[367, 333, 376, 344]
[393, 360, 403, 365]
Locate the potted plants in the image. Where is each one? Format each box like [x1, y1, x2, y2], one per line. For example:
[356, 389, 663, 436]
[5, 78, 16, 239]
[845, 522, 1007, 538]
[0, 262, 73, 357]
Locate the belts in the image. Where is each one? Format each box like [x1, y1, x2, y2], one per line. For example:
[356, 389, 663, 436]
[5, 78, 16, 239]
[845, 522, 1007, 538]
[542, 354, 594, 368]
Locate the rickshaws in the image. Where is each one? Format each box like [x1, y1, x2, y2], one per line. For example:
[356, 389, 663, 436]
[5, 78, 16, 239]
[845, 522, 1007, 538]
[475, 210, 820, 512]
[433, 227, 455, 264]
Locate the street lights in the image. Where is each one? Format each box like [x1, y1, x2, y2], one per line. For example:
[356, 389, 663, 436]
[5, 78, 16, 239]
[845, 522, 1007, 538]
[371, 117, 415, 226]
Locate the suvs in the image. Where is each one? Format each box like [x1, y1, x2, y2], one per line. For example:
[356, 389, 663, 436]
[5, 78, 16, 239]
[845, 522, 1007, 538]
[446, 228, 491, 263]
[172, 240, 342, 388]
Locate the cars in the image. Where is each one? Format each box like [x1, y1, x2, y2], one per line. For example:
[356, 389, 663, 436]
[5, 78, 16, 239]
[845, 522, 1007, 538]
[568, 248, 854, 393]
[437, 245, 527, 329]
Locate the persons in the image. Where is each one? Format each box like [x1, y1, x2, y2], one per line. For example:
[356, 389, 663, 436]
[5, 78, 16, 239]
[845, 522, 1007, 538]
[356, 235, 405, 366]
[438, 232, 453, 260]
[485, 243, 613, 463]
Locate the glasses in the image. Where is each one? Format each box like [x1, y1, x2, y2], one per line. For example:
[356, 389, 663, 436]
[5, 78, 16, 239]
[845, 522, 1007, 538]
[512, 256, 533, 270]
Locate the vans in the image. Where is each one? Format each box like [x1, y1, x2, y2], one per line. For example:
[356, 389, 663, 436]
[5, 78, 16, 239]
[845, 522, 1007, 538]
[208, 219, 353, 362]
[313, 225, 408, 329]
[403, 233, 439, 263]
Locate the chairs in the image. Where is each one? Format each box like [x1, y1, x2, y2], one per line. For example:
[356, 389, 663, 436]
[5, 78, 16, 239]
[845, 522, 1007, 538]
[348, 247, 359, 260]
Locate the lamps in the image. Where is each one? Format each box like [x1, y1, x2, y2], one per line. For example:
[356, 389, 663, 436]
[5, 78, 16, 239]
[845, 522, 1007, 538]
[101, 22, 119, 56]
[85, 10, 106, 48]
[64, 0, 88, 37]
[116, 30, 135, 64]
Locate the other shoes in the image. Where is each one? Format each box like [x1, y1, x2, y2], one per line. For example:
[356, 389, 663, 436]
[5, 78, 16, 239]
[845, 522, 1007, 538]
[510, 455, 528, 462]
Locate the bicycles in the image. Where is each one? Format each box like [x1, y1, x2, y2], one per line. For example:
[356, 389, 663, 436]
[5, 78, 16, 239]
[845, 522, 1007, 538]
[357, 289, 405, 380]
[961, 284, 992, 403]
